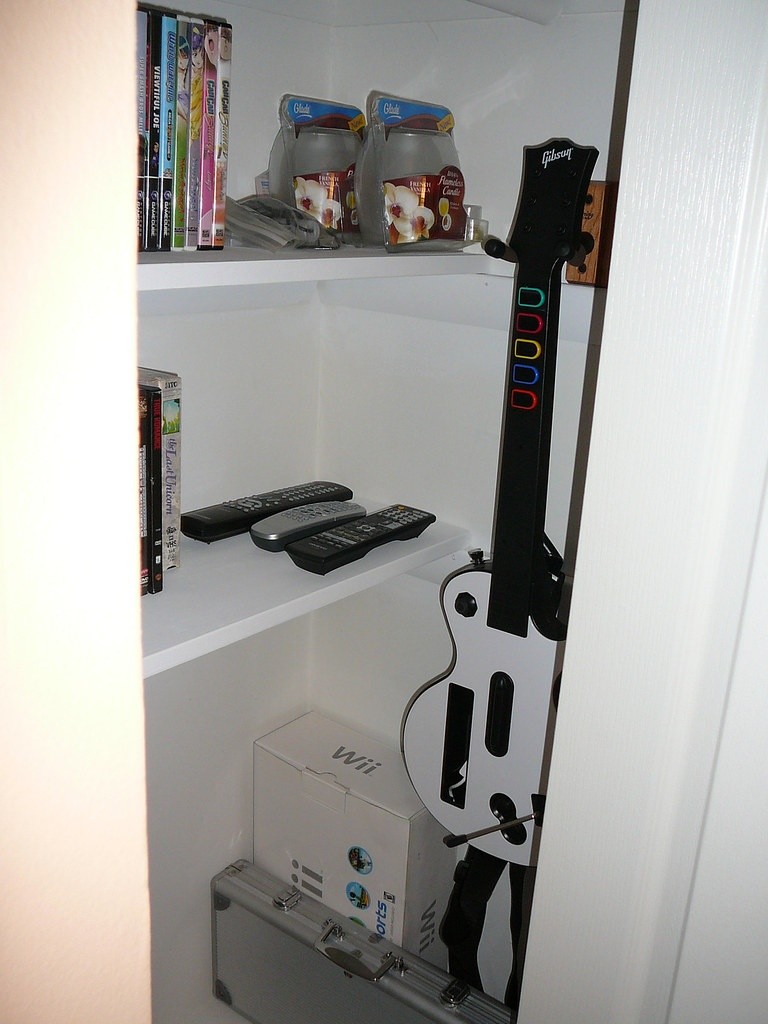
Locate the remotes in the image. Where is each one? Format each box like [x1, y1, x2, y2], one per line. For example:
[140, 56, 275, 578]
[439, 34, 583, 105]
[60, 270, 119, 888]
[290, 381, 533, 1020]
[250, 501, 366, 552]
[180, 481, 352, 544]
[285, 504, 437, 576]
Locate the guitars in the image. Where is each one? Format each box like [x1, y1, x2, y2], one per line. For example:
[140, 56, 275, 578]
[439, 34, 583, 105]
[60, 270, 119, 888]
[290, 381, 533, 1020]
[399, 135, 603, 868]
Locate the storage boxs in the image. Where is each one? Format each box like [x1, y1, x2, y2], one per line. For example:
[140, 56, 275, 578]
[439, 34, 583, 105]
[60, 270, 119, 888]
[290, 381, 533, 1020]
[254, 711, 454, 975]
[210, 859, 510, 1024]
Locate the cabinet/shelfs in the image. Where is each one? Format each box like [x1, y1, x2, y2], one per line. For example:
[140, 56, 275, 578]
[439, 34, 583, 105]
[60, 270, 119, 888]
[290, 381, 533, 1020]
[0, 0, 642, 1024]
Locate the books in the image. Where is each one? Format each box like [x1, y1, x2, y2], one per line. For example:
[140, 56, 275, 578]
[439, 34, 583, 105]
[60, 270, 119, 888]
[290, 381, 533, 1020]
[139, 366, 181, 596]
